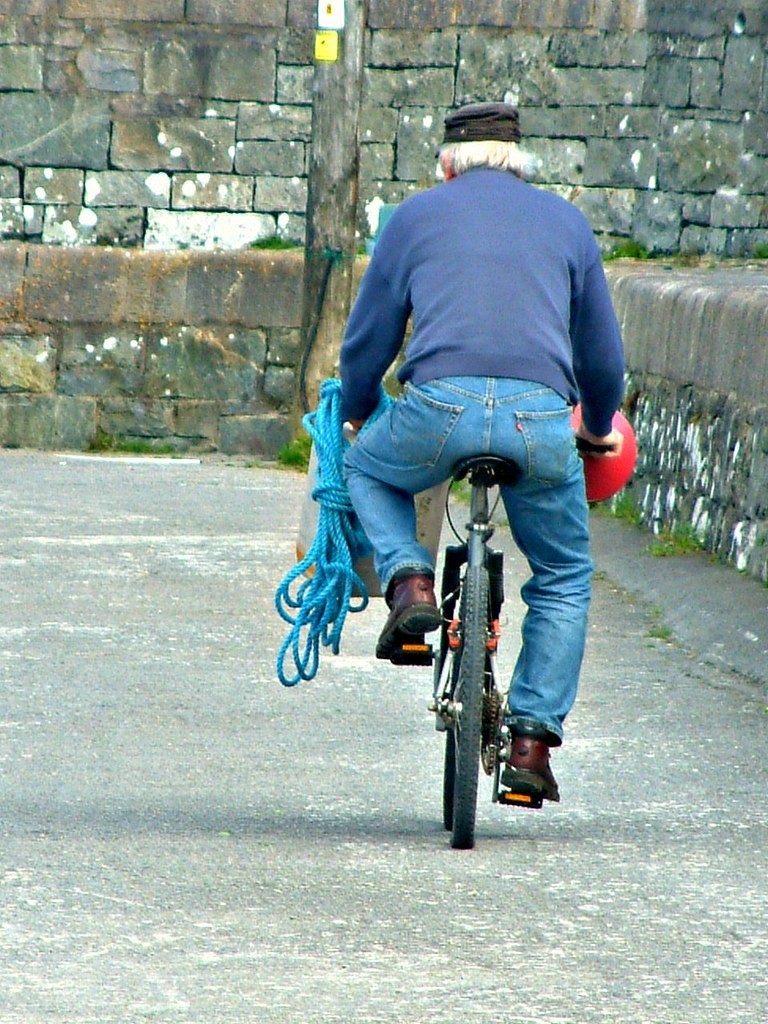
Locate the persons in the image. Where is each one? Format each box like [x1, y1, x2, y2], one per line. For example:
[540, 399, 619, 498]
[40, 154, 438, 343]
[340, 101, 627, 801]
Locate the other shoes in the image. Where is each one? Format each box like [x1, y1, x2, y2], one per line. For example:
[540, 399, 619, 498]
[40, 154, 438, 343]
[502, 728, 560, 802]
[376, 575, 440, 659]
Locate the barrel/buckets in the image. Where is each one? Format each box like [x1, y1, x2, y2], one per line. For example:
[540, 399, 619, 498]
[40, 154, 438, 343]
[295, 422, 453, 600]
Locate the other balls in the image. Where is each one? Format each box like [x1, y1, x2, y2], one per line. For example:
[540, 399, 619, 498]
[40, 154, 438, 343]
[569, 402, 639, 507]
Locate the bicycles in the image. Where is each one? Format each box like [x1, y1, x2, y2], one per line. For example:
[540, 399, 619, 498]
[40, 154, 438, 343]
[357, 425, 615, 850]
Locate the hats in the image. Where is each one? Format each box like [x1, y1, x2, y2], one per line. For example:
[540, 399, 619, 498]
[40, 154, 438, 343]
[432, 102, 521, 160]
